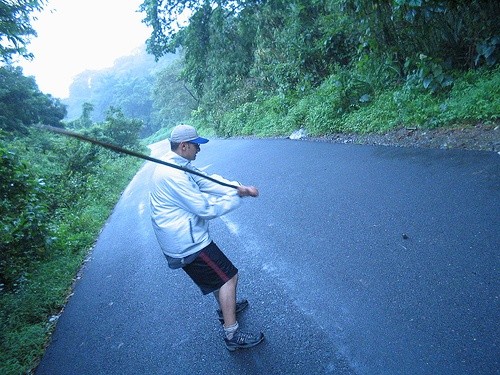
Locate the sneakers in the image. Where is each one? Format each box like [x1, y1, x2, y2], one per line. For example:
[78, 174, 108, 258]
[215, 299, 248, 323]
[222, 330, 264, 351]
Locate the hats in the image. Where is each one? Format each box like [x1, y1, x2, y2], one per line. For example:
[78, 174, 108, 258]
[167, 123, 209, 144]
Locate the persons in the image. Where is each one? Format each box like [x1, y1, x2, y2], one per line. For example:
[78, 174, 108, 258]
[148, 126, 265, 351]
